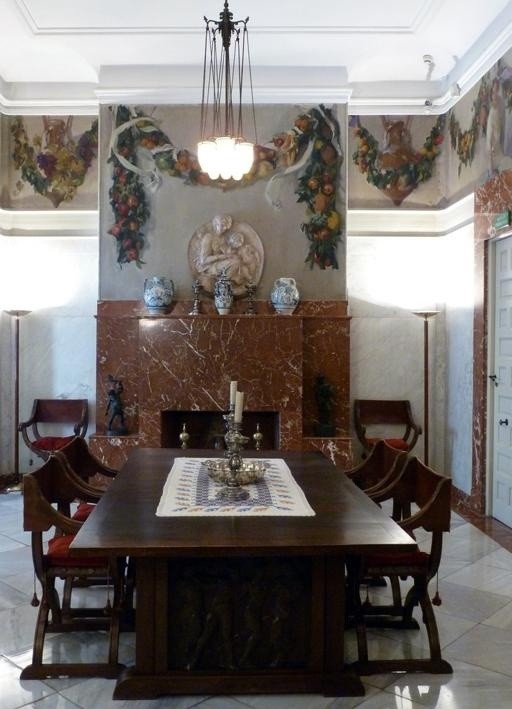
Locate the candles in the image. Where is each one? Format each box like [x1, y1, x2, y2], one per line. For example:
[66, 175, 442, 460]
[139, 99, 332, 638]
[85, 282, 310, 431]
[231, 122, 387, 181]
[234, 391, 244, 423]
[229, 381, 238, 404]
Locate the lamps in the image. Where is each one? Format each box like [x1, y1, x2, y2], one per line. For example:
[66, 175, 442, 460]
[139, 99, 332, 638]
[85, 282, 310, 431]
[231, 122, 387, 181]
[197, 0, 255, 182]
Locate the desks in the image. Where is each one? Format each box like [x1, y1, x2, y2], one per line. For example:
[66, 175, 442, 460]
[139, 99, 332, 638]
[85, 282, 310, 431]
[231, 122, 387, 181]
[68, 448, 419, 701]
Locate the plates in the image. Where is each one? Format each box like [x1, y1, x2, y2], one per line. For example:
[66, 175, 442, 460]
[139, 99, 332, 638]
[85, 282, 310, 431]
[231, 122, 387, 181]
[200, 459, 269, 485]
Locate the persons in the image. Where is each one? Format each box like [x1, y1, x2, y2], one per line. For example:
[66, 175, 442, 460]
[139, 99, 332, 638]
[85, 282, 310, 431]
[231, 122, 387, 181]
[197, 214, 256, 285]
[103, 381, 125, 430]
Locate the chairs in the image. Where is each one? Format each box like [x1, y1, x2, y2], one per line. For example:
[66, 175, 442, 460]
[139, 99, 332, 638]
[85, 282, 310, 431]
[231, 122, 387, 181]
[354, 399, 422, 459]
[346, 455, 454, 673]
[20, 455, 136, 681]
[17, 399, 88, 463]
[56, 436, 136, 618]
[344, 439, 407, 616]
